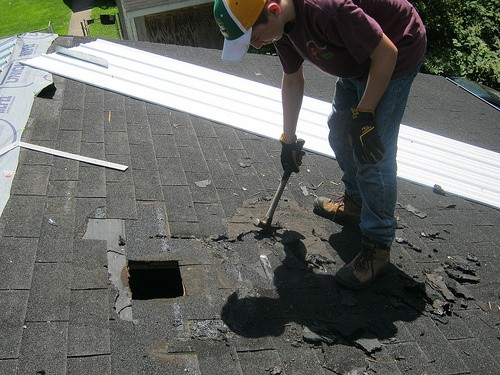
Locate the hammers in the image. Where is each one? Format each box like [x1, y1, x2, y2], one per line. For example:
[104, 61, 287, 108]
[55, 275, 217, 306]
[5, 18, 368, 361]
[253, 139, 306, 232]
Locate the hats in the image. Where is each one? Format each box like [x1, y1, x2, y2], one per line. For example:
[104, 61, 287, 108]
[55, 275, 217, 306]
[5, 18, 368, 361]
[213, 0, 268, 62]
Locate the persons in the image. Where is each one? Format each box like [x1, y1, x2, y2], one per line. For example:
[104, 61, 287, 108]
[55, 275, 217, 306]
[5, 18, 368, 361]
[212, 0, 428, 289]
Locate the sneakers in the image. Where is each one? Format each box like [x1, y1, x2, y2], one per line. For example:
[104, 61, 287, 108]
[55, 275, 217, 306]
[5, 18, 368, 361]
[314, 196, 361, 228]
[341, 236, 390, 286]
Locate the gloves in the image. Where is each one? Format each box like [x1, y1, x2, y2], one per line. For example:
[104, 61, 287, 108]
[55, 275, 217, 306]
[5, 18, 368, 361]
[347, 108, 384, 165]
[280, 132, 305, 173]
